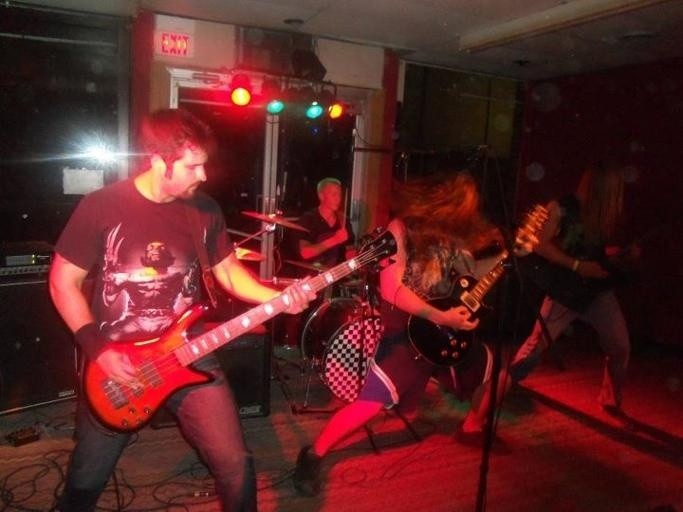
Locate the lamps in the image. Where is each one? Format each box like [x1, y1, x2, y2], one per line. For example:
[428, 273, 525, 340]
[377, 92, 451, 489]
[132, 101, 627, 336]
[228, 68, 343, 122]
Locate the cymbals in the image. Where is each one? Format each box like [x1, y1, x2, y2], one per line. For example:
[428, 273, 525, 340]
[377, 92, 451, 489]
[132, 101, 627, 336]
[234, 248, 266, 261]
[240, 210, 310, 232]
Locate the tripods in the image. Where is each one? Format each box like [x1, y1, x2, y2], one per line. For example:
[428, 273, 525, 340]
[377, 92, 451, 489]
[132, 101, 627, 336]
[300, 286, 427, 452]
[254, 121, 305, 414]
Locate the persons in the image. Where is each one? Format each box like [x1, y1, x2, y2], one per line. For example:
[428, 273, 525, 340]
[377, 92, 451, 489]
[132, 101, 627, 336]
[290, 167, 518, 500]
[46, 106, 320, 512]
[452, 156, 642, 443]
[296, 173, 355, 272]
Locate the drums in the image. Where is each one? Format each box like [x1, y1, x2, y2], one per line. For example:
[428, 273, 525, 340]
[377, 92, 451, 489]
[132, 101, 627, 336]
[301, 294, 385, 404]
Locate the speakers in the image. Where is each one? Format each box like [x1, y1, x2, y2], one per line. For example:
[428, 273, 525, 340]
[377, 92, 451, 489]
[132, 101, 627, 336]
[153, 332, 273, 428]
[1, 280, 80, 414]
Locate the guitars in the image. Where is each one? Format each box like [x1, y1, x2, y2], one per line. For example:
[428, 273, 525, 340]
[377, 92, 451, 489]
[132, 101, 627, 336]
[407, 205, 549, 367]
[77, 227, 396, 433]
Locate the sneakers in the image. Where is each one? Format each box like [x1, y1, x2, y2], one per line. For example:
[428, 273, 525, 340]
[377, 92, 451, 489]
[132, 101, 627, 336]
[294, 446, 323, 495]
[507, 381, 532, 396]
[602, 405, 625, 418]
[455, 431, 508, 453]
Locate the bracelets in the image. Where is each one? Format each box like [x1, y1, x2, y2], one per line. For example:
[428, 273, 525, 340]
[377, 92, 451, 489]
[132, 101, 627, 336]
[74, 322, 110, 363]
[569, 258, 580, 274]
